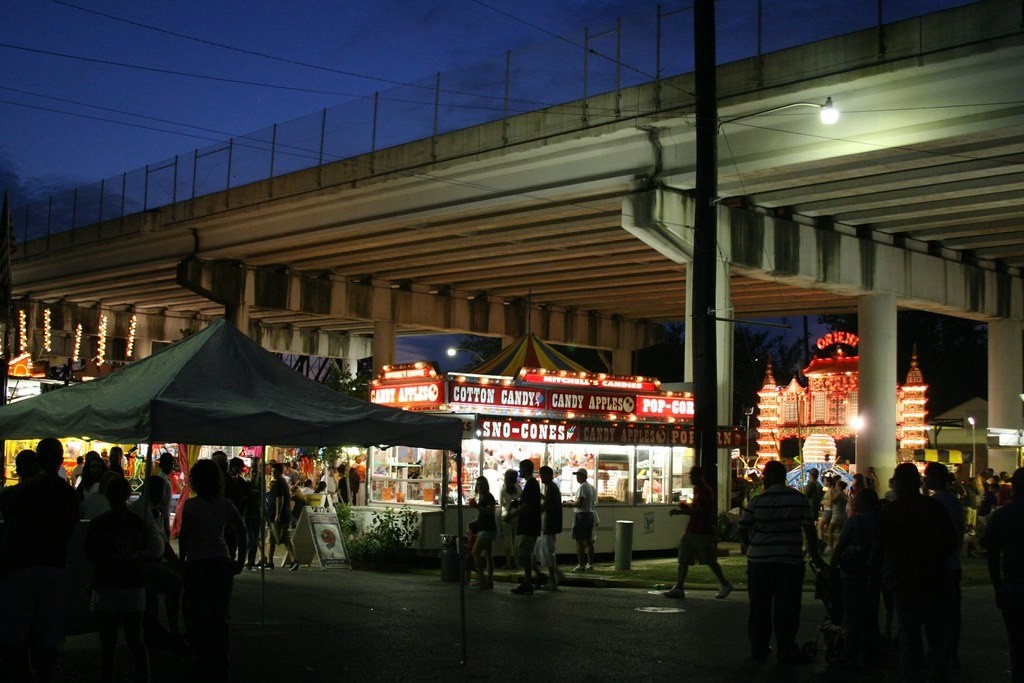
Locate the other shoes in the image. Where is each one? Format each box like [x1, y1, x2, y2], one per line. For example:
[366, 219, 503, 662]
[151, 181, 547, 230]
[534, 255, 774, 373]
[535, 573, 547, 588]
[573, 562, 593, 574]
[288, 559, 299, 571]
[258, 562, 274, 571]
[502, 562, 519, 570]
[663, 585, 685, 598]
[511, 582, 533, 595]
[241, 563, 256, 572]
[715, 581, 733, 599]
[469, 580, 493, 590]
[756, 645, 773, 658]
[541, 577, 558, 591]
[825, 546, 833, 553]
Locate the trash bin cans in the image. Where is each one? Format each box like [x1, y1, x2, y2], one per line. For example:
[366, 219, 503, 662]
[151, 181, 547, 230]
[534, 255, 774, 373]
[440, 534, 472, 583]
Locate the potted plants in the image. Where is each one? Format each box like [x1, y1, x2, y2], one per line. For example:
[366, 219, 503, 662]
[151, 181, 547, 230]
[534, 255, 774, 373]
[338, 505, 420, 573]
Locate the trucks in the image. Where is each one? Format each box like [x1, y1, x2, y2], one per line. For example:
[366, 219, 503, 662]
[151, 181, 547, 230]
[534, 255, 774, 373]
[371, 367, 697, 553]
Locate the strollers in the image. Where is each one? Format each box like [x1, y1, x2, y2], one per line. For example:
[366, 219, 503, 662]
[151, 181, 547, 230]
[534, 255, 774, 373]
[797, 548, 882, 664]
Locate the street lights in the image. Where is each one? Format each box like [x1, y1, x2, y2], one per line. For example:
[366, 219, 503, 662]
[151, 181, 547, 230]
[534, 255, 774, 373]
[849, 415, 865, 464]
[687, 93, 840, 553]
[968, 417, 976, 478]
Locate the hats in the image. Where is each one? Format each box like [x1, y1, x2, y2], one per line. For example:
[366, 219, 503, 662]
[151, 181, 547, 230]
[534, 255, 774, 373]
[573, 469, 587, 476]
[159, 453, 176, 468]
[806, 468, 819, 477]
[686, 466, 704, 476]
[1004, 467, 1024, 483]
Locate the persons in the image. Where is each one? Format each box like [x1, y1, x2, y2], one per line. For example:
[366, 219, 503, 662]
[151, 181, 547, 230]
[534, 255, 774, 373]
[663, 464, 733, 599]
[210, 451, 366, 571]
[1, 439, 248, 683]
[468, 459, 598, 593]
[739, 459, 1023, 683]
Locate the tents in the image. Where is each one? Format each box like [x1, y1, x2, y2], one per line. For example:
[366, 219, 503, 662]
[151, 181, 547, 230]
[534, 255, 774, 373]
[0, 318, 469, 663]
